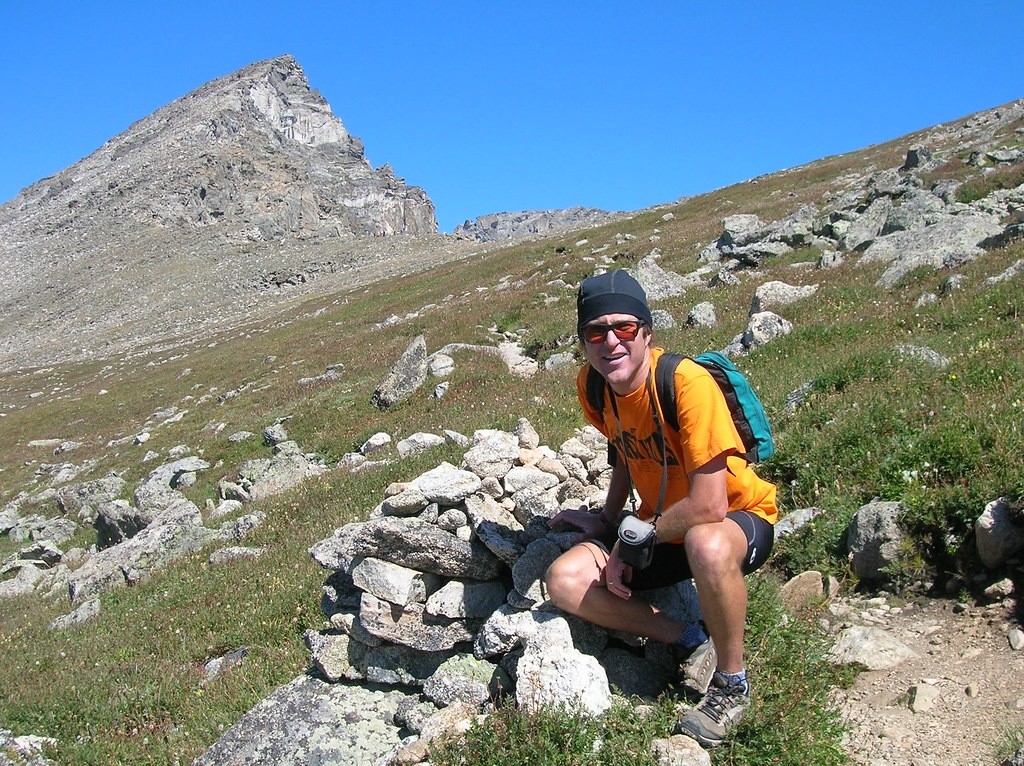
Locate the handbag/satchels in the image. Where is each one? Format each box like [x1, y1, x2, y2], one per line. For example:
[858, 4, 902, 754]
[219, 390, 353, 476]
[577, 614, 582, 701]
[618, 514, 656, 569]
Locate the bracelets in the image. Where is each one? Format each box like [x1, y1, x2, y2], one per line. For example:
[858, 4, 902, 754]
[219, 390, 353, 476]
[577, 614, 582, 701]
[599, 511, 612, 528]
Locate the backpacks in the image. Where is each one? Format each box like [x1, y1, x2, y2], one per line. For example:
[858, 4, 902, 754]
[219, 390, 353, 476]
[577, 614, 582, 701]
[587, 351, 775, 468]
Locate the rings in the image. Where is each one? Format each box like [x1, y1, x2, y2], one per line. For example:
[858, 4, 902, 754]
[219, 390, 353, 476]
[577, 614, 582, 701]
[609, 581, 614, 585]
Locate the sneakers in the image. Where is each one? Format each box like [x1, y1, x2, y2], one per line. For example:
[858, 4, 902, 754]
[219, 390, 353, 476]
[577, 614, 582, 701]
[675, 619, 719, 698]
[678, 672, 751, 748]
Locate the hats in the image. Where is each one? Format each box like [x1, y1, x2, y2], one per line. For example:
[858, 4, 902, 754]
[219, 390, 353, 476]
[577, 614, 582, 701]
[577, 270, 652, 330]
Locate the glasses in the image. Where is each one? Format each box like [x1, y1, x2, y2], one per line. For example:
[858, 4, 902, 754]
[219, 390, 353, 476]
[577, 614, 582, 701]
[579, 320, 646, 344]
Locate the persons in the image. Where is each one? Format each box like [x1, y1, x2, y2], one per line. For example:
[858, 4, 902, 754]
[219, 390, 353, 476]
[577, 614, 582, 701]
[545, 269, 779, 748]
